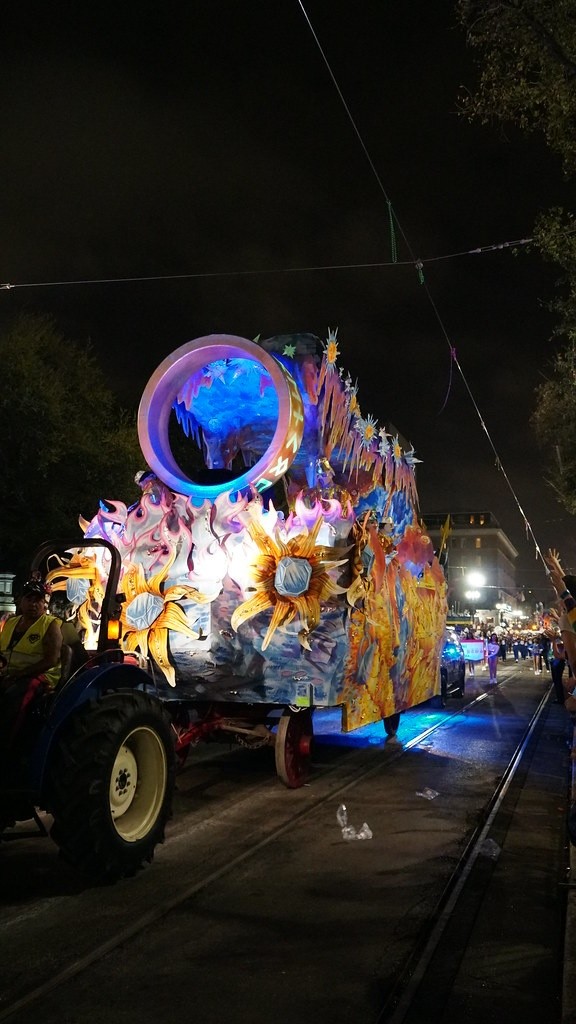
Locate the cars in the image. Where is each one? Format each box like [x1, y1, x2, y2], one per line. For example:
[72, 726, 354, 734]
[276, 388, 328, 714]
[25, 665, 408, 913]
[437, 628, 465, 707]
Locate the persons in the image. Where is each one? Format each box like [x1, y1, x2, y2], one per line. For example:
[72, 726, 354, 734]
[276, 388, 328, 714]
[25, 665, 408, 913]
[456, 547, 576, 710]
[134, 471, 172, 505]
[0, 579, 63, 717]
[306, 459, 353, 520]
[360, 511, 394, 572]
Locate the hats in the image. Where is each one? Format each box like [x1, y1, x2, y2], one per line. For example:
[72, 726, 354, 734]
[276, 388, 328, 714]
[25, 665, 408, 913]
[23, 581, 46, 596]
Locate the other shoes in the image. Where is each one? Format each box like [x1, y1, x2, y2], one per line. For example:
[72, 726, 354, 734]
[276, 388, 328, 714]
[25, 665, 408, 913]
[493, 678, 498, 683]
[488, 679, 493, 684]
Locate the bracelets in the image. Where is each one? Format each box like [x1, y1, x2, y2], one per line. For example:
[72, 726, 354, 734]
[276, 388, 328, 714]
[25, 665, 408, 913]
[560, 590, 570, 599]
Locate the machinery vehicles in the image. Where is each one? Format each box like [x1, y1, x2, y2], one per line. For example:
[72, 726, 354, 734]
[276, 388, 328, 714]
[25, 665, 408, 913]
[0, 327, 451, 877]
